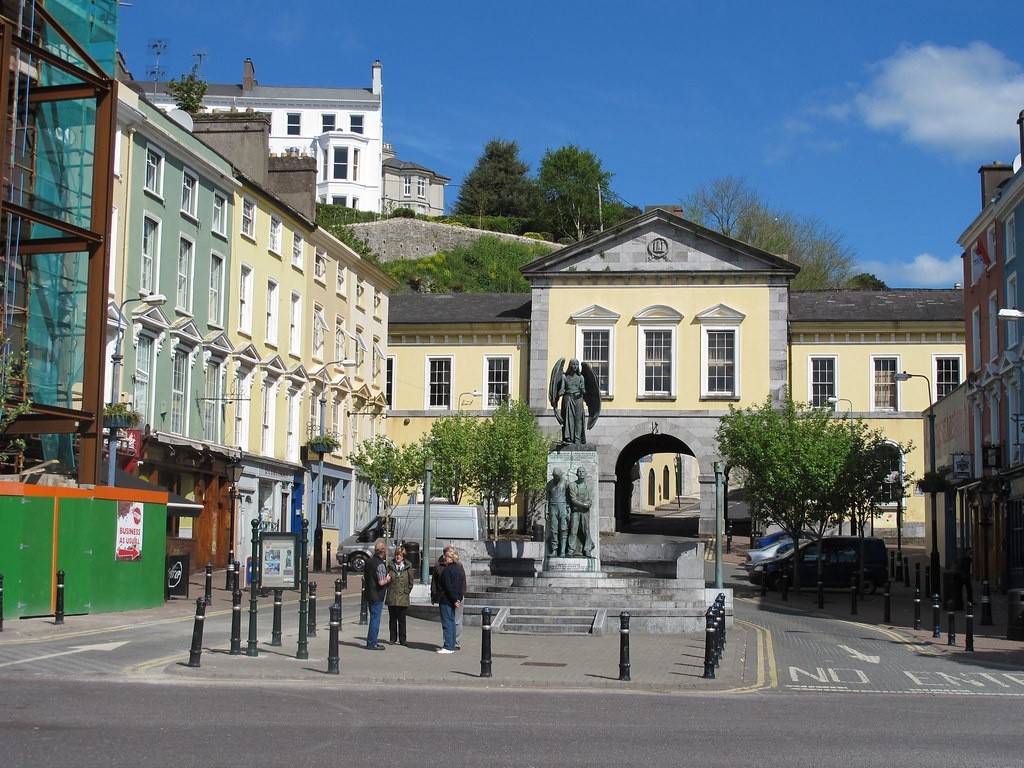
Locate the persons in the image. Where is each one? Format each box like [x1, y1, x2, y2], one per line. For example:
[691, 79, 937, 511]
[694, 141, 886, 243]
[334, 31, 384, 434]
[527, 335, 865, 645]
[365, 542, 414, 650]
[544, 466, 595, 556]
[430, 546, 467, 655]
[548, 356, 602, 444]
[954, 547, 978, 607]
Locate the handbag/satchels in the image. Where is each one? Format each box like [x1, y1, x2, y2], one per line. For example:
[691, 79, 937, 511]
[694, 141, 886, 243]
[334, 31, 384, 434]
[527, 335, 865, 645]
[432, 591, 444, 605]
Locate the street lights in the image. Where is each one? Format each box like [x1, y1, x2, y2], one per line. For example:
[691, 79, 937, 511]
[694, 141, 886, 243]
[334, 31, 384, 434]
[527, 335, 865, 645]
[894, 372, 941, 599]
[312, 360, 356, 574]
[225, 453, 246, 592]
[828, 397, 858, 535]
[457, 390, 483, 412]
[108, 293, 168, 490]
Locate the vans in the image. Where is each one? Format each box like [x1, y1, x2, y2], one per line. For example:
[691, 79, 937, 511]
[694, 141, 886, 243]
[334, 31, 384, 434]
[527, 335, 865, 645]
[746, 534, 890, 596]
[336, 507, 487, 573]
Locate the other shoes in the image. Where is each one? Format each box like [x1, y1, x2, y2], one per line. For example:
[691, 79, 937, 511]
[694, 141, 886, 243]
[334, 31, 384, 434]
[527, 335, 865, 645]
[435, 647, 454, 654]
[453, 647, 460, 650]
[367, 644, 385, 650]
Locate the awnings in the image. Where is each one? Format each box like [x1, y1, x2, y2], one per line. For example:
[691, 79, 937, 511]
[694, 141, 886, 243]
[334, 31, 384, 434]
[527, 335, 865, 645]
[154, 431, 244, 462]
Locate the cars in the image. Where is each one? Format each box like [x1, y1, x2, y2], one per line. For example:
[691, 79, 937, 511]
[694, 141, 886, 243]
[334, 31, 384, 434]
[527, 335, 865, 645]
[753, 530, 821, 549]
[745, 538, 830, 566]
